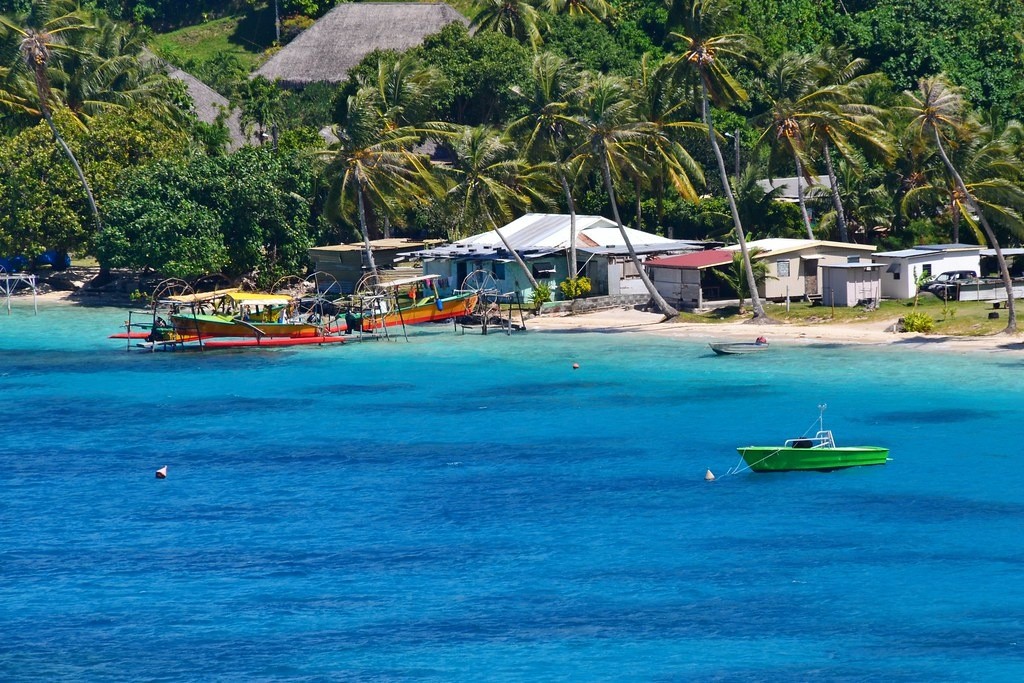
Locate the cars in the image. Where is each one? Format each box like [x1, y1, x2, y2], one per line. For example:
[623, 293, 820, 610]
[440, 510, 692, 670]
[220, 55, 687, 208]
[38, 250, 71, 268]
[0, 255, 40, 289]
[196, 263, 250, 288]
[219, 256, 266, 277]
[149, 279, 192, 296]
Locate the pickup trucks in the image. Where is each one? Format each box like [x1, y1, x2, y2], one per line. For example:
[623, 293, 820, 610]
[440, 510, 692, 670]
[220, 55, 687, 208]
[919, 271, 1001, 301]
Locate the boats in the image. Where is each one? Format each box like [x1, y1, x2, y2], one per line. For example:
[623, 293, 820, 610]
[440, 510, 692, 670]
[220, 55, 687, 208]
[150, 288, 288, 343]
[336, 274, 483, 330]
[707, 337, 770, 355]
[737, 404, 891, 472]
[169, 293, 328, 338]
